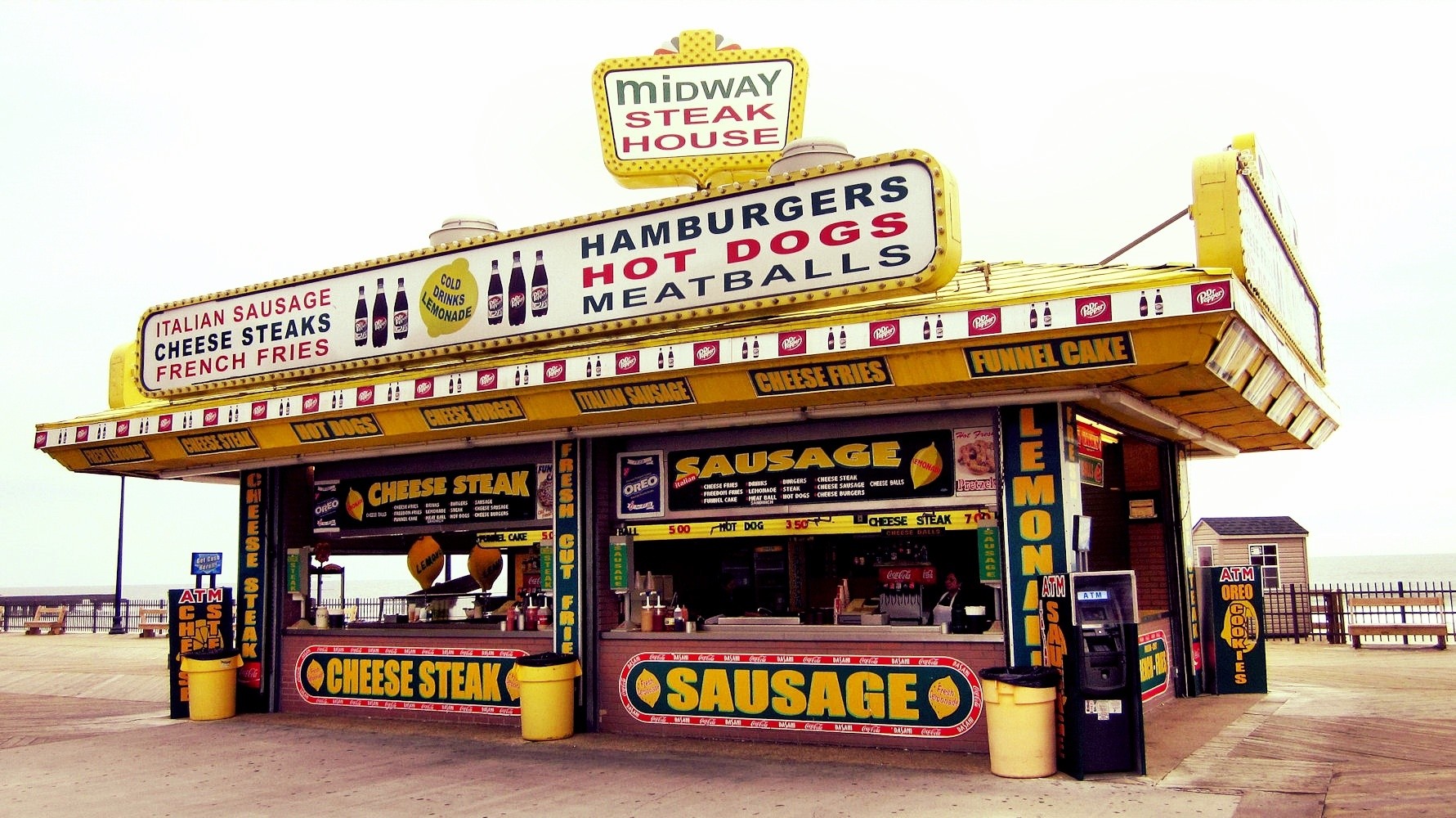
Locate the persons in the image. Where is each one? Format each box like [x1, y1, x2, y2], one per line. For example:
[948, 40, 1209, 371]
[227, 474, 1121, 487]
[928, 571, 968, 625]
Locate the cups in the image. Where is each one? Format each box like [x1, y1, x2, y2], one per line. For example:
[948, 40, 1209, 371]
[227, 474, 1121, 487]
[500, 621, 506, 631]
[834, 579, 850, 625]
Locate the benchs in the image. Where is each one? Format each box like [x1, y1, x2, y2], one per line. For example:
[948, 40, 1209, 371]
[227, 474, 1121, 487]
[24, 605, 69, 634]
[1345, 592, 1449, 650]
[138, 606, 170, 637]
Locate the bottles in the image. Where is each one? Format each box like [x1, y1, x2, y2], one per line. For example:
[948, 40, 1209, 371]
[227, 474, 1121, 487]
[506, 605, 524, 631]
[641, 595, 688, 632]
[408, 603, 420, 622]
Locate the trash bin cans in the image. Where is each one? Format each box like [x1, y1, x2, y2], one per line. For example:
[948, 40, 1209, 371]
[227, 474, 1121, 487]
[516, 653, 583, 742]
[184, 649, 244, 721]
[980, 666, 1060, 778]
